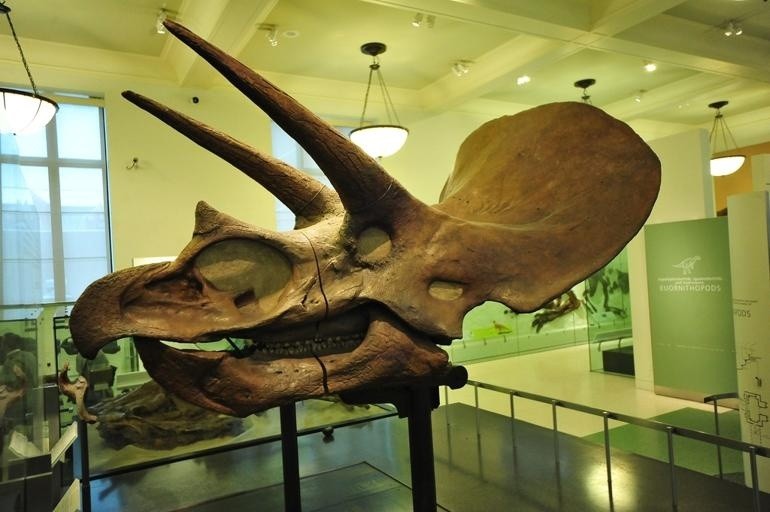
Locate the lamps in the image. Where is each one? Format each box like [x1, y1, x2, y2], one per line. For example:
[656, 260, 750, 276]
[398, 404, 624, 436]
[266, 28, 280, 47]
[451, 61, 469, 78]
[154, 6, 172, 35]
[723, 20, 743, 38]
[348, 44, 409, 160]
[412, 13, 424, 29]
[710, 101, 746, 177]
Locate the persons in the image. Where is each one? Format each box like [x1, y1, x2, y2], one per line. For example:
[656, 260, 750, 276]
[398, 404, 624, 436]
[0, 331, 37, 434]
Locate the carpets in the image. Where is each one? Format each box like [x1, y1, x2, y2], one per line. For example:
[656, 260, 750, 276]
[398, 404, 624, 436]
[579, 407, 744, 482]
